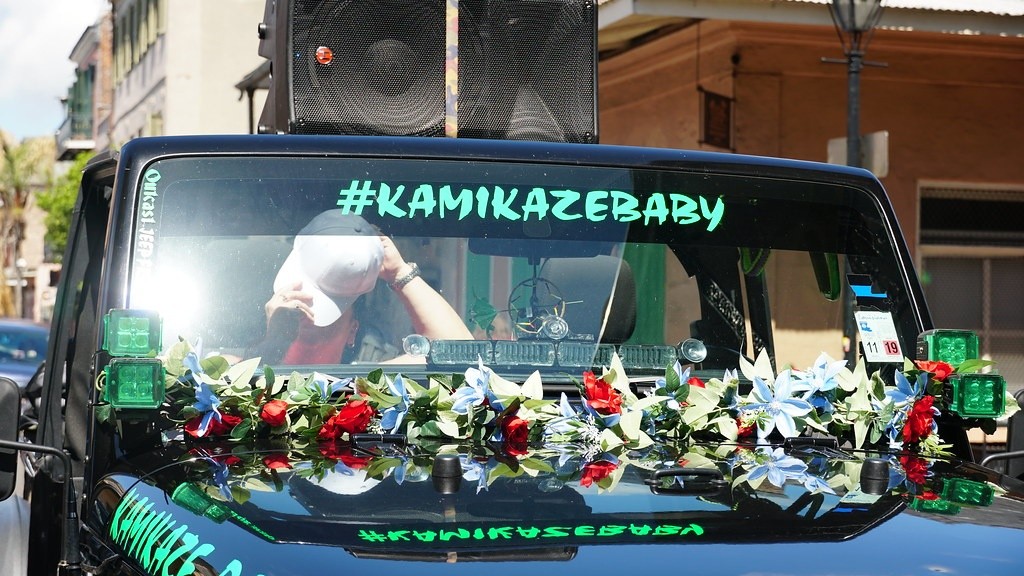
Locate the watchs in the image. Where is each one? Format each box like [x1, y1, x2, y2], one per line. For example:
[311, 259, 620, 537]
[388, 260, 421, 292]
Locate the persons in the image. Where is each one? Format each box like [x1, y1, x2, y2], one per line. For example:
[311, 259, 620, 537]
[226, 223, 475, 366]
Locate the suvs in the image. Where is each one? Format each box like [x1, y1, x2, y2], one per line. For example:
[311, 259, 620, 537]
[0, 134, 1024, 576]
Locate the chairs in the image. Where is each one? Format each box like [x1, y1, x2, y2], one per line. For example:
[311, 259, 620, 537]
[527, 250, 636, 357]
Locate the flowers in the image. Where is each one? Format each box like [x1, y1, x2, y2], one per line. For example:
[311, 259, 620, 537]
[153, 343, 974, 508]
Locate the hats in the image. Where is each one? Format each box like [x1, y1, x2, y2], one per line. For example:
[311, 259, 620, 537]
[272, 207, 382, 327]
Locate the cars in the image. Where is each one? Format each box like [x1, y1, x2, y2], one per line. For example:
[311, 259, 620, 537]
[1, 320, 65, 422]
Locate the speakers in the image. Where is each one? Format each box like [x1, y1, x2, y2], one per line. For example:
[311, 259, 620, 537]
[257, 0, 599, 144]
